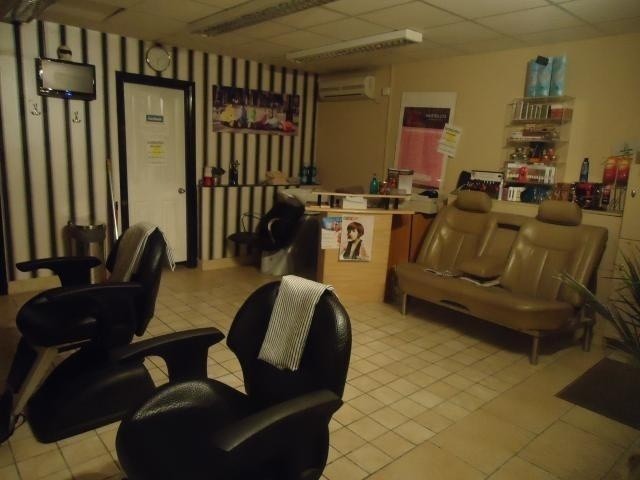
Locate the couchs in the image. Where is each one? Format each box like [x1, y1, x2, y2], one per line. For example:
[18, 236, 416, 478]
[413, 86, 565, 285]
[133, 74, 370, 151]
[396, 191, 608, 366]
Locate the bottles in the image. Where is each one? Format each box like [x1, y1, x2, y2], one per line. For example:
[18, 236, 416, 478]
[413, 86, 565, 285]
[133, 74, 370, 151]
[302, 163, 316, 184]
[387, 172, 397, 195]
[378, 176, 388, 195]
[370, 178, 379, 193]
[512, 145, 556, 161]
[600, 188, 610, 210]
[509, 174, 551, 185]
[579, 158, 589, 183]
[466, 178, 500, 194]
[569, 184, 577, 203]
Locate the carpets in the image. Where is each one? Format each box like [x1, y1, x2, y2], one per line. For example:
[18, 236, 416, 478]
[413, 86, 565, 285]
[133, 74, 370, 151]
[554, 356, 640, 432]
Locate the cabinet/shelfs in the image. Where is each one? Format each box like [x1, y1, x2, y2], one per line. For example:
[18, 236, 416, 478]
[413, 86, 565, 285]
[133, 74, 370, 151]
[505, 95, 575, 183]
[304, 190, 415, 214]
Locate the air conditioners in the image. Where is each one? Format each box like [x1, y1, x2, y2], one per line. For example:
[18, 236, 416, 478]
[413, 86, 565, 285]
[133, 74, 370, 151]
[317, 75, 375, 101]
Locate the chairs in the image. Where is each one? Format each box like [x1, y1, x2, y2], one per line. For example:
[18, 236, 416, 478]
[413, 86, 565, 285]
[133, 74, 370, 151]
[8, 220, 165, 443]
[110, 275, 352, 480]
[229, 192, 304, 266]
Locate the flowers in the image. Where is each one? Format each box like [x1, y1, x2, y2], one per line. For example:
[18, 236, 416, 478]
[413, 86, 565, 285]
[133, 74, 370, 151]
[213, 167, 225, 177]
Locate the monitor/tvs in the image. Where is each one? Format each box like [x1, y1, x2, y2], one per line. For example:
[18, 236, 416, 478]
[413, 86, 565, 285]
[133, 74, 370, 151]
[37, 57, 96, 101]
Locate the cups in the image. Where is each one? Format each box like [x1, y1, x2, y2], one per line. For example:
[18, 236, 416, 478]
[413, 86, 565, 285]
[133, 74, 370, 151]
[199, 177, 212, 186]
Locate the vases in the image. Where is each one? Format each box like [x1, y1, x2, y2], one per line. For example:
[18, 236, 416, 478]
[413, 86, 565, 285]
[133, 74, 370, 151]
[212, 176, 221, 186]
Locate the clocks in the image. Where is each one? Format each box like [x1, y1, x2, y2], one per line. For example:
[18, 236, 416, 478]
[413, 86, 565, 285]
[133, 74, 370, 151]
[146, 45, 170, 73]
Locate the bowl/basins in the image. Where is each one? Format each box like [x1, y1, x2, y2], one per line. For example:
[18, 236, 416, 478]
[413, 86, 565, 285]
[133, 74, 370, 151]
[511, 131, 522, 139]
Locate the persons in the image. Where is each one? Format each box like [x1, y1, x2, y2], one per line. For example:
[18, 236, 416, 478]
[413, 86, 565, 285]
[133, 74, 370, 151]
[339, 222, 370, 261]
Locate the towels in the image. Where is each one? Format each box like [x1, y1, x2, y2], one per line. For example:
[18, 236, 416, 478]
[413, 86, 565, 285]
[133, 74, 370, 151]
[257, 275, 339, 374]
[108, 221, 175, 285]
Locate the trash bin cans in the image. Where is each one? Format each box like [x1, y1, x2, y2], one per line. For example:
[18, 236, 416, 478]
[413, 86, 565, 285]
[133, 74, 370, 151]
[68, 219, 106, 285]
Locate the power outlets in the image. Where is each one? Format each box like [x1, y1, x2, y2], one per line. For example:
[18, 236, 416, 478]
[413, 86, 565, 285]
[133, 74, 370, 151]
[382, 87, 390, 96]
